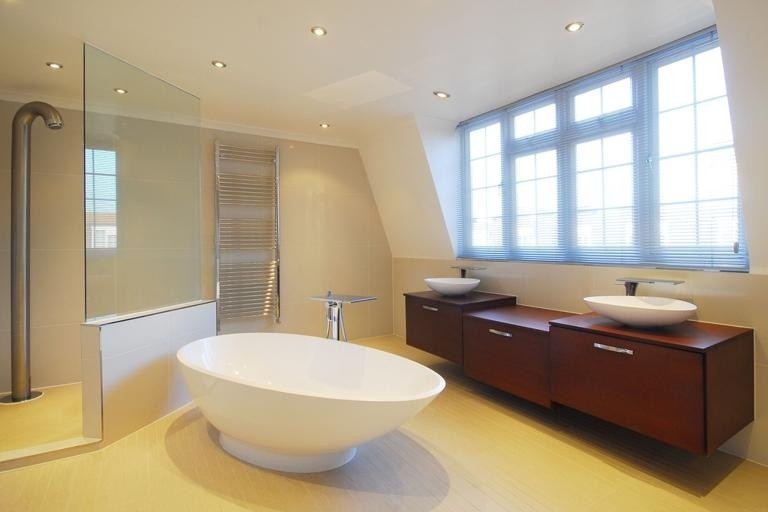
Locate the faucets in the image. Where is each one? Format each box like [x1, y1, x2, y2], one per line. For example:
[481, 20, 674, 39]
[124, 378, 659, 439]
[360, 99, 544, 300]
[451, 267, 467, 278]
[614, 281, 639, 296]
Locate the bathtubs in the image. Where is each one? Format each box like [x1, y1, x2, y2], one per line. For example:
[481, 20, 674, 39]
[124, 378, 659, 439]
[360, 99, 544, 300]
[175, 332, 447, 474]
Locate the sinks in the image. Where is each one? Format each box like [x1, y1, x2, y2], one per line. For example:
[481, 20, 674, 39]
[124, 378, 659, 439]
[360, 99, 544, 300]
[583, 296, 698, 330]
[422, 277, 481, 297]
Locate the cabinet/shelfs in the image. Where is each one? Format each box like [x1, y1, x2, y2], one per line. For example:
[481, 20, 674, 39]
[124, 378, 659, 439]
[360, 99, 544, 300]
[544, 312, 755, 455]
[462, 303, 580, 413]
[403, 288, 516, 363]
